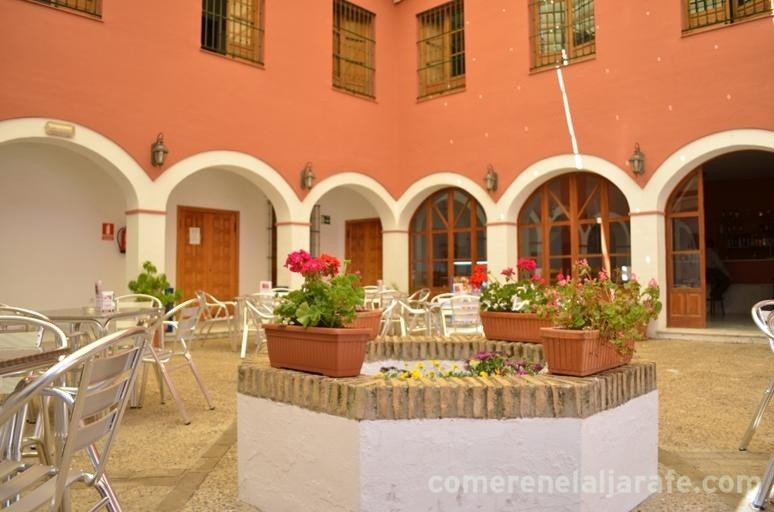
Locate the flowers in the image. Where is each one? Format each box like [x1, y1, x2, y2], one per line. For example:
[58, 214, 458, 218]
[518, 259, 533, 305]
[271, 250, 365, 327]
[468, 258, 545, 312]
[546, 258, 666, 363]
[607, 270, 663, 328]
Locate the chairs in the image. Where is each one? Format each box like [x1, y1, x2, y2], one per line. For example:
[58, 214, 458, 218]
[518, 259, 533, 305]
[740, 300, 774, 510]
[0, 281, 538, 512]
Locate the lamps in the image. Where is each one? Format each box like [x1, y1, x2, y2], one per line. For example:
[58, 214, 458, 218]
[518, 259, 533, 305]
[628, 143, 645, 174]
[151, 132, 168, 166]
[482, 163, 496, 190]
[303, 162, 315, 189]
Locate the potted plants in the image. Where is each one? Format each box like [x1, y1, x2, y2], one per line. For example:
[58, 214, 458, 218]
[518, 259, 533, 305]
[479, 310, 558, 344]
[540, 326, 635, 376]
[631, 317, 651, 340]
[129, 262, 185, 350]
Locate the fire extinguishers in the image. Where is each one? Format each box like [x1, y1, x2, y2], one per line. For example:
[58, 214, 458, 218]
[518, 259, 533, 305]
[117, 225, 126, 253]
